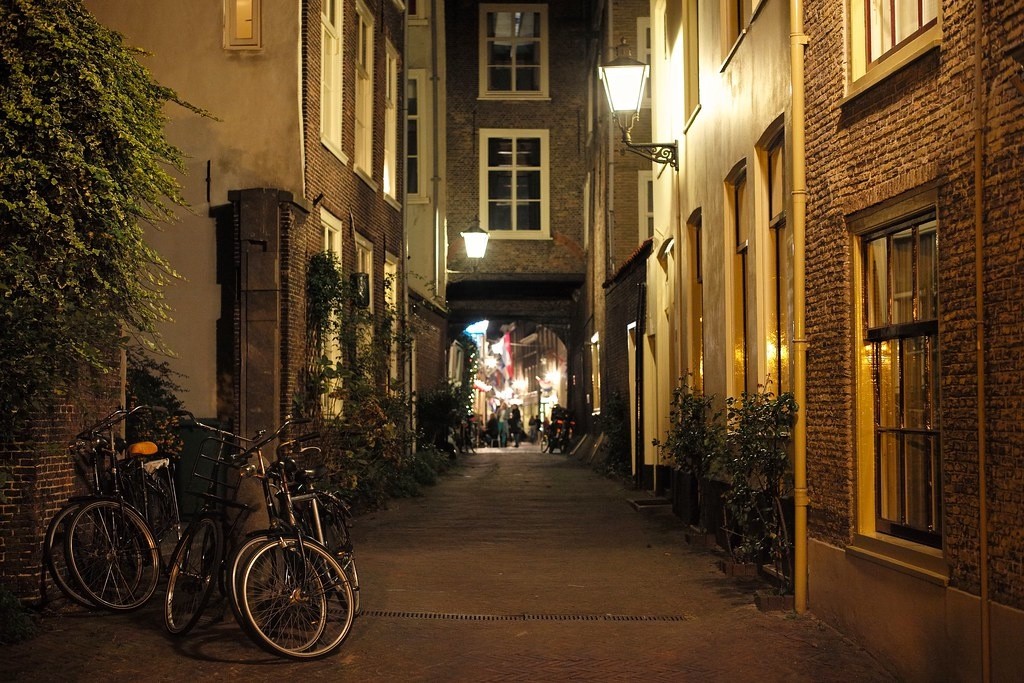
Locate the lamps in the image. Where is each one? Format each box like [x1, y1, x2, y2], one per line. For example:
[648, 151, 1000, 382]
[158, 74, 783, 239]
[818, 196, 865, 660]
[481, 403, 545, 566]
[599, 37, 679, 172]
[447, 214, 490, 258]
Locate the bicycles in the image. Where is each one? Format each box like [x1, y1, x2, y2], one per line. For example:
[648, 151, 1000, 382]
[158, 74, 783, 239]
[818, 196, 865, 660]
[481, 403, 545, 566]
[456, 414, 489, 455]
[43, 402, 361, 660]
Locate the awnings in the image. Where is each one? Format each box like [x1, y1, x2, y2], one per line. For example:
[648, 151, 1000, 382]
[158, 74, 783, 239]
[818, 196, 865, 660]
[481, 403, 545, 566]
[521, 391, 557, 404]
[472, 380, 492, 392]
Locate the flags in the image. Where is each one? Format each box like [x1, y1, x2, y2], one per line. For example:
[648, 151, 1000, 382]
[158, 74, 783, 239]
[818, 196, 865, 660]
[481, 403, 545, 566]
[498, 335, 514, 379]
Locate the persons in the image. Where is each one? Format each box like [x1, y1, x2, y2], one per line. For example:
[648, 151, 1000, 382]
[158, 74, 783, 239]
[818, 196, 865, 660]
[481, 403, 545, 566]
[478, 399, 550, 448]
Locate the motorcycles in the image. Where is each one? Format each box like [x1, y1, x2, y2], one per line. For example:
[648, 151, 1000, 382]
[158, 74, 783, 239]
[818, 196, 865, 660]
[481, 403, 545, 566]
[539, 409, 577, 455]
